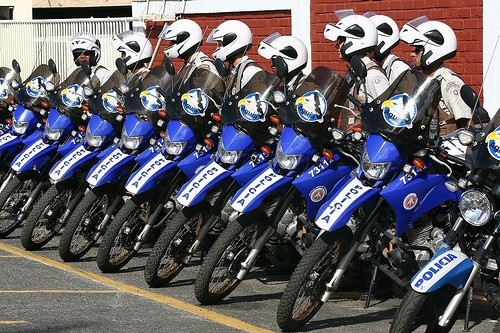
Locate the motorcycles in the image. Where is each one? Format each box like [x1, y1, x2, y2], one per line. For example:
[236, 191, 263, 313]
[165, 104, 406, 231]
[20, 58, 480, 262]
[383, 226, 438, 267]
[0, 55, 500, 333]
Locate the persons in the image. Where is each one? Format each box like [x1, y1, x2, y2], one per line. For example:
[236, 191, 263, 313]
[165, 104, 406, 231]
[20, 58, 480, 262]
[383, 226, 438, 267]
[101, 29, 162, 238]
[32, 31, 110, 225]
[210, 8, 500, 333]
[134, 17, 226, 249]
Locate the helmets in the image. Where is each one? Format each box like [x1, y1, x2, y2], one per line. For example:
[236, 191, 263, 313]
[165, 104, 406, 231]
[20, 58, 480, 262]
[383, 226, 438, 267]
[211, 20, 252, 62]
[162, 19, 202, 59]
[118, 34, 153, 70]
[71, 35, 101, 66]
[400, 21, 457, 69]
[324, 15, 378, 58]
[369, 14, 400, 57]
[258, 36, 308, 75]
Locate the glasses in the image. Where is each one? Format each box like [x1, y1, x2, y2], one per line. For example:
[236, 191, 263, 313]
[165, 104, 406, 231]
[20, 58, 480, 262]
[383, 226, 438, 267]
[74, 51, 91, 57]
